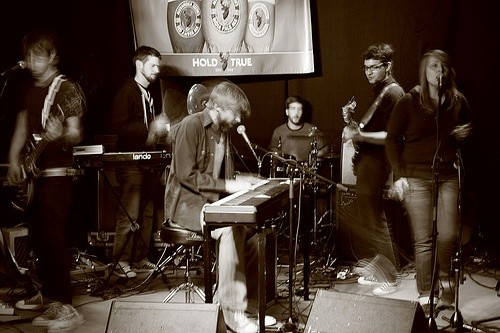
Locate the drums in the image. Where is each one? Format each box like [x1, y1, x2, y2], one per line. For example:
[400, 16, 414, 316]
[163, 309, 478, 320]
[257, 152, 339, 193]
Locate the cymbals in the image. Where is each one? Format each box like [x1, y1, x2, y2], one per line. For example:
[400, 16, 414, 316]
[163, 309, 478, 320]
[317, 153, 338, 161]
[287, 129, 339, 138]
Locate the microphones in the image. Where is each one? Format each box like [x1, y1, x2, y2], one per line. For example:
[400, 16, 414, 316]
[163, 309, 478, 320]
[237, 124, 258, 160]
[0, 60, 28, 77]
[436, 73, 446, 95]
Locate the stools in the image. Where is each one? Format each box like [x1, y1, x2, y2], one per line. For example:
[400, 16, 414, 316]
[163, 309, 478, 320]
[160, 220, 212, 304]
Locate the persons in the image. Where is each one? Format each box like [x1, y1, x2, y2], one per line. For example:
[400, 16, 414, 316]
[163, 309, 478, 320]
[9, 38, 87, 330]
[339, 44, 413, 288]
[163, 81, 284, 331]
[268, 96, 329, 168]
[101, 46, 171, 278]
[389, 48, 472, 316]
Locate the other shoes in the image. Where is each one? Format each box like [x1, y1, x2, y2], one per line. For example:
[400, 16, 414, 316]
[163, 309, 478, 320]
[357, 276, 384, 286]
[373, 283, 399, 296]
[422, 303, 435, 315]
[263, 313, 276, 327]
[224, 309, 258, 333]
[441, 285, 456, 305]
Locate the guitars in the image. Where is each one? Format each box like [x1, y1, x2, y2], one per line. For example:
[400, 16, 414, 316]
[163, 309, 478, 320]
[340, 95, 361, 185]
[1, 104, 64, 212]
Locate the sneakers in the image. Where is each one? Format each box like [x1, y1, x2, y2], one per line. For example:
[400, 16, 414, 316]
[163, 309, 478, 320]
[15, 291, 55, 309]
[48, 307, 86, 332]
[133, 262, 165, 273]
[112, 266, 137, 278]
[31, 302, 70, 327]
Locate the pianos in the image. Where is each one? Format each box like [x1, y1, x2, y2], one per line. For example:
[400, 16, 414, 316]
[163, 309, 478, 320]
[203, 177, 310, 333]
[68, 142, 174, 276]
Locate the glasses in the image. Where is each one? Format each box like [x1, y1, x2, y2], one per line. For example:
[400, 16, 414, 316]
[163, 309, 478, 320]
[361, 63, 386, 72]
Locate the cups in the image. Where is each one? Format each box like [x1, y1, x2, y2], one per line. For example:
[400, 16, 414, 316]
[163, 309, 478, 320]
[244, 0, 276, 53]
[167, 0, 205, 53]
[201, 0, 249, 53]
